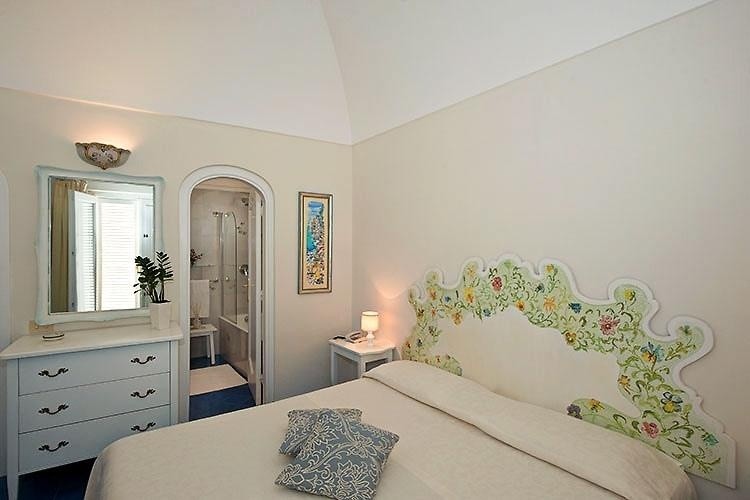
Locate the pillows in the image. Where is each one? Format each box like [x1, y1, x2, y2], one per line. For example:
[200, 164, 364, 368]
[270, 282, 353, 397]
[273, 407, 400, 500]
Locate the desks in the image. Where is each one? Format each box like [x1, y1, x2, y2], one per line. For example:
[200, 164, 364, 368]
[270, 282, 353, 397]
[327, 337, 396, 385]
[190, 323, 219, 365]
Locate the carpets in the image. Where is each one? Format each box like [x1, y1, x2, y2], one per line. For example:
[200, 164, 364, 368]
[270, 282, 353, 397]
[189, 363, 248, 396]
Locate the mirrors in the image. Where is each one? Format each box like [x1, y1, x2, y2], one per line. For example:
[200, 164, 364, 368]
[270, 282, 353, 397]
[34, 165, 164, 326]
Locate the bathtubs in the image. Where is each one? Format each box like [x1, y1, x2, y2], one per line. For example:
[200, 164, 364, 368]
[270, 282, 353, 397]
[219, 313, 248, 381]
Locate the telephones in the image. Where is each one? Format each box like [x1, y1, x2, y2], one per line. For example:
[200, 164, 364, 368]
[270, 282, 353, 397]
[346, 331, 367, 343]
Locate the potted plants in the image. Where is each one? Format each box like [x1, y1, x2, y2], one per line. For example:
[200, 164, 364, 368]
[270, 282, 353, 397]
[134, 250, 174, 330]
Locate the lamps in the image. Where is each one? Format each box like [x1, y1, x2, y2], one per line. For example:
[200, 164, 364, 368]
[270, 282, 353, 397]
[75, 142, 132, 171]
[361, 310, 380, 346]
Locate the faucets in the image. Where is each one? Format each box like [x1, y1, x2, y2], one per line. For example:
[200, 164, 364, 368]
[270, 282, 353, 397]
[245, 316, 249, 321]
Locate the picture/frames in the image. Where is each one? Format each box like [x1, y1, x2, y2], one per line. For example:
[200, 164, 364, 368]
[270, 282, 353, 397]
[297, 192, 333, 294]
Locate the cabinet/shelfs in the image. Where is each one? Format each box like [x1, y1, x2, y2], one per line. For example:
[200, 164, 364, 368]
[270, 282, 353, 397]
[0, 320, 184, 500]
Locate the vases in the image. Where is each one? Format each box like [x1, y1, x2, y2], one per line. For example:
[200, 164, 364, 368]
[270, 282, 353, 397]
[192, 314, 201, 329]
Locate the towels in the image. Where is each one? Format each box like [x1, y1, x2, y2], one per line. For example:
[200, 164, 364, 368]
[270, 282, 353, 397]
[190, 279, 209, 318]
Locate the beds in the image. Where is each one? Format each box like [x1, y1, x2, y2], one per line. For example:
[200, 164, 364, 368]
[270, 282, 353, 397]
[84, 248, 737, 500]
